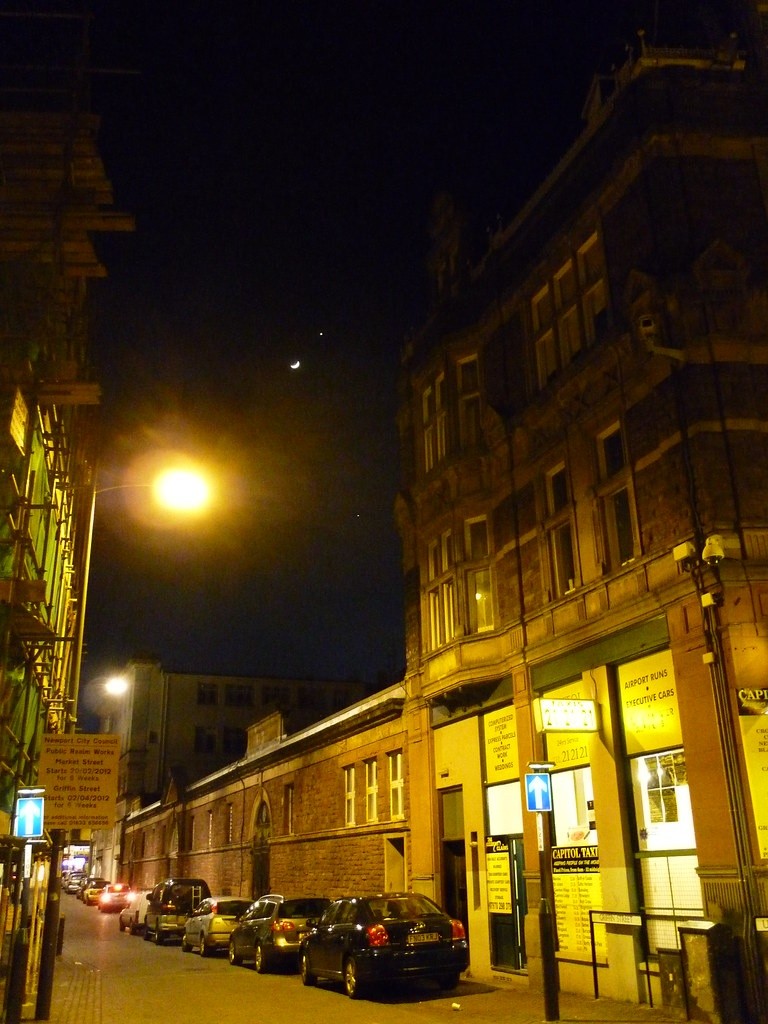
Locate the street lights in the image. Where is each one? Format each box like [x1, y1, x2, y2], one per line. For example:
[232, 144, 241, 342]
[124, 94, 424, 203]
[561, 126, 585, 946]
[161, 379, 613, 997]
[36, 465, 215, 1024]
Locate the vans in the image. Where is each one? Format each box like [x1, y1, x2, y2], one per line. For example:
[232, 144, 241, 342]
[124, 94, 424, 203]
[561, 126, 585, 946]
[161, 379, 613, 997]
[144, 878, 211, 945]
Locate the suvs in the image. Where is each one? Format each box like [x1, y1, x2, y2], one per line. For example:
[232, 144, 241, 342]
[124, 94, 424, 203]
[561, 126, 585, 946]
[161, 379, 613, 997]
[227, 895, 332, 974]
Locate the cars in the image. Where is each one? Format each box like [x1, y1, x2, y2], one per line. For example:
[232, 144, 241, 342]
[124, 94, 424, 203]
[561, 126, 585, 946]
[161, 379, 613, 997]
[62, 869, 84, 889]
[296, 891, 469, 998]
[76, 878, 105, 901]
[99, 882, 133, 913]
[118, 890, 154, 935]
[66, 873, 87, 894]
[83, 880, 111, 906]
[182, 897, 256, 959]
